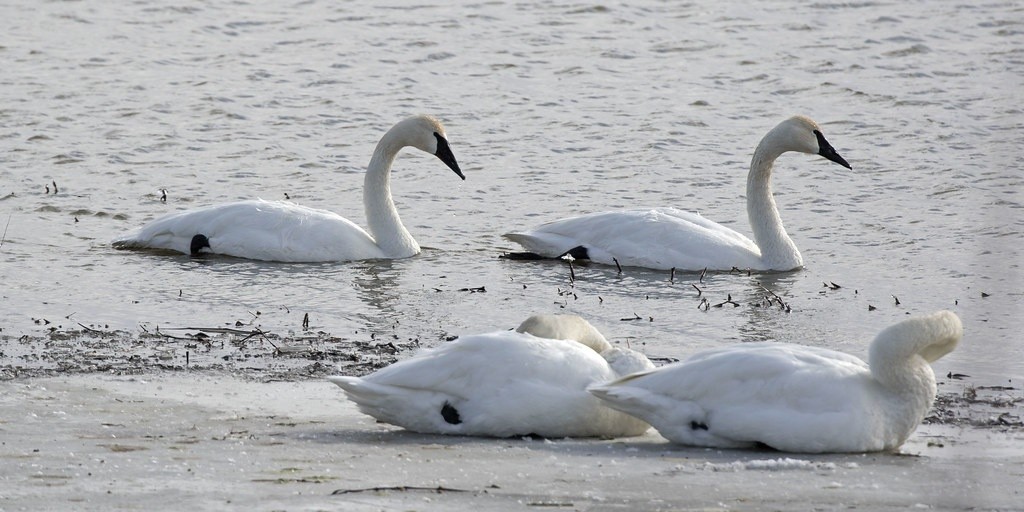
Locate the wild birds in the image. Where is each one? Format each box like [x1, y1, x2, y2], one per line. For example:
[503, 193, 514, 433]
[587, 309, 961, 453]
[112, 115, 465, 265]
[327, 315, 657, 437]
[502, 115, 853, 272]
